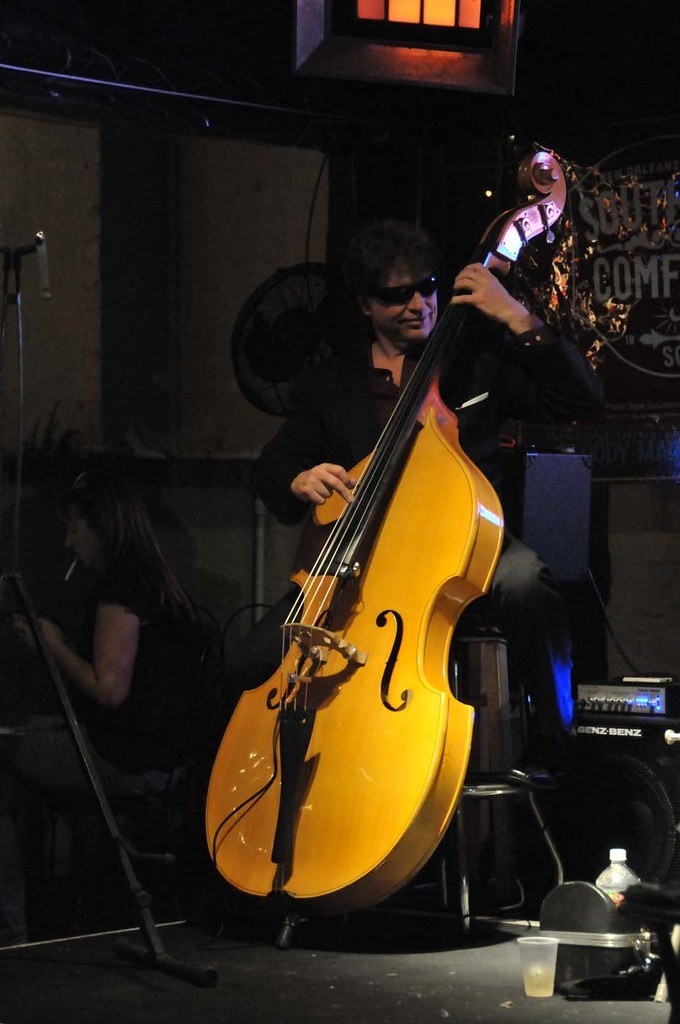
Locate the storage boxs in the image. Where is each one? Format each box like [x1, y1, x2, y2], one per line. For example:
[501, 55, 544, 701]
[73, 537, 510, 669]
[539, 880, 662, 1001]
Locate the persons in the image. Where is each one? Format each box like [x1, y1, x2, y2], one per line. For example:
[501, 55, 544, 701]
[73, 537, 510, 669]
[221, 220, 603, 796]
[0, 470, 227, 952]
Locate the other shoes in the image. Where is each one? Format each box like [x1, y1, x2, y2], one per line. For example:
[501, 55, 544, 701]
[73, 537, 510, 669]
[0, 926, 28, 955]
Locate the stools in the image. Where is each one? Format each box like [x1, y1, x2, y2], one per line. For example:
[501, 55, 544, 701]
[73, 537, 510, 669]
[442, 630, 563, 931]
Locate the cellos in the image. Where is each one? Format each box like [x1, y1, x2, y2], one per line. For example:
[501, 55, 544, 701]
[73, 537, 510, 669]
[205, 151, 567, 949]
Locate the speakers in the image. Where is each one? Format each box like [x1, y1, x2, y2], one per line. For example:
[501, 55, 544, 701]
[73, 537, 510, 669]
[510, 711, 680, 921]
[499, 451, 593, 583]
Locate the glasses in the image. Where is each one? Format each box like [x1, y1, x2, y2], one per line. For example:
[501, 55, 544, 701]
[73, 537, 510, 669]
[360, 271, 437, 306]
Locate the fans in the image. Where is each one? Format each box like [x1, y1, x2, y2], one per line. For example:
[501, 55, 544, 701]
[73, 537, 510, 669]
[230, 262, 346, 418]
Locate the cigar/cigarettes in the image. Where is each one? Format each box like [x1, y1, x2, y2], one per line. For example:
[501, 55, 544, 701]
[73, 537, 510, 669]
[64, 555, 79, 581]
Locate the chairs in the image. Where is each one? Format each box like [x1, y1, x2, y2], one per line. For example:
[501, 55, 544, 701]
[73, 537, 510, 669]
[41, 674, 229, 928]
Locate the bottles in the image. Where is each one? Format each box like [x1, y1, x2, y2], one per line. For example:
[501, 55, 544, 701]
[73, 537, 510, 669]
[594, 847, 643, 908]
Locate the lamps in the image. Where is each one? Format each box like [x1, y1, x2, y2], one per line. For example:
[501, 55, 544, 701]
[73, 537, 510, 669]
[294, 0, 518, 97]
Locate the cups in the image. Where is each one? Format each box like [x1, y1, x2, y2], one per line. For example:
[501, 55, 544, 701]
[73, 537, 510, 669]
[515, 936, 560, 998]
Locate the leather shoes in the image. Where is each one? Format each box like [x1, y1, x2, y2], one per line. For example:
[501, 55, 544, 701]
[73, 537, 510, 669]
[506, 745, 579, 787]
